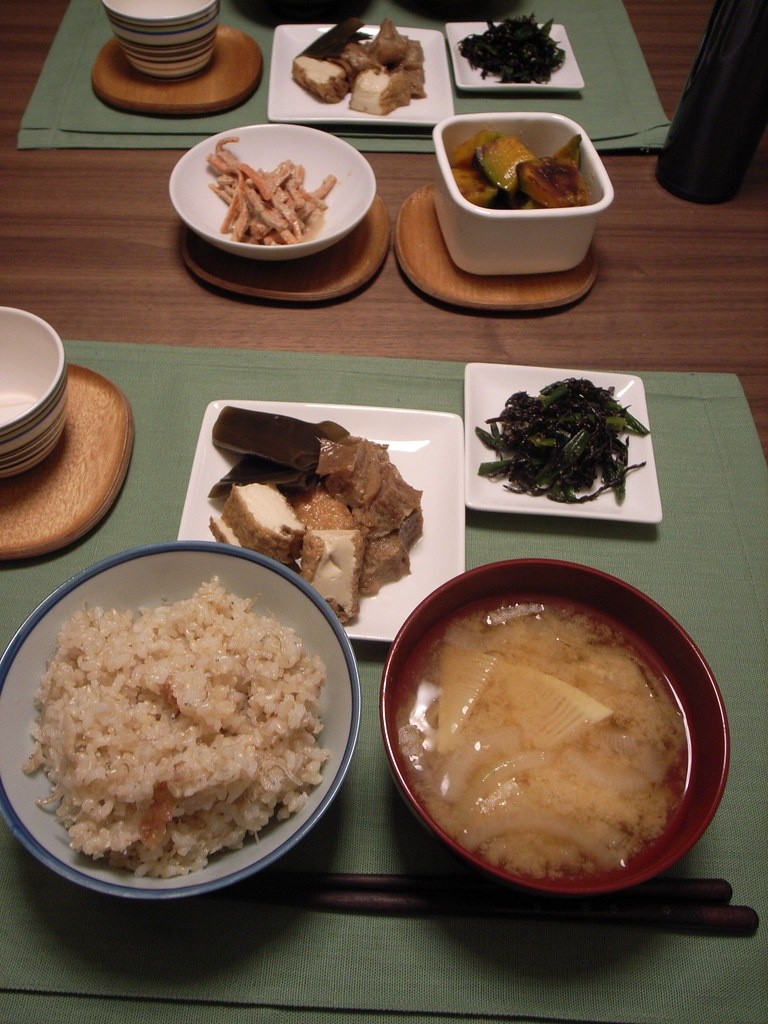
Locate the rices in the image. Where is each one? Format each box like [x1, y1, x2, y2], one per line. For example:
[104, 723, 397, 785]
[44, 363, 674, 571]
[22, 574, 330, 879]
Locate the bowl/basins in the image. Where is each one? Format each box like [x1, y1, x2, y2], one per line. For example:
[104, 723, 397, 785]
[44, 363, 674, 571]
[430, 111, 615, 277]
[379, 558, 731, 899]
[168, 124, 377, 261]
[0, 539, 362, 901]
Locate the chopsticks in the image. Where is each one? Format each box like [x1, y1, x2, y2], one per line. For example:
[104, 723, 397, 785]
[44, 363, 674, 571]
[281, 871, 760, 936]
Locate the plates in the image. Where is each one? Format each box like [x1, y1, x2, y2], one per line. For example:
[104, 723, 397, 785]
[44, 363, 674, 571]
[463, 360, 663, 526]
[267, 22, 455, 127]
[444, 21, 585, 93]
[177, 399, 466, 644]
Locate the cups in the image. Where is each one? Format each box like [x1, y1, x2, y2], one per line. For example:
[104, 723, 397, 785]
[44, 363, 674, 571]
[0, 305, 69, 480]
[653, 0, 768, 205]
[100, 0, 221, 79]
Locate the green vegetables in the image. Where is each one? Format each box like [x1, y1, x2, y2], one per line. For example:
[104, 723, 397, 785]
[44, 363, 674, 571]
[475, 386, 649, 502]
